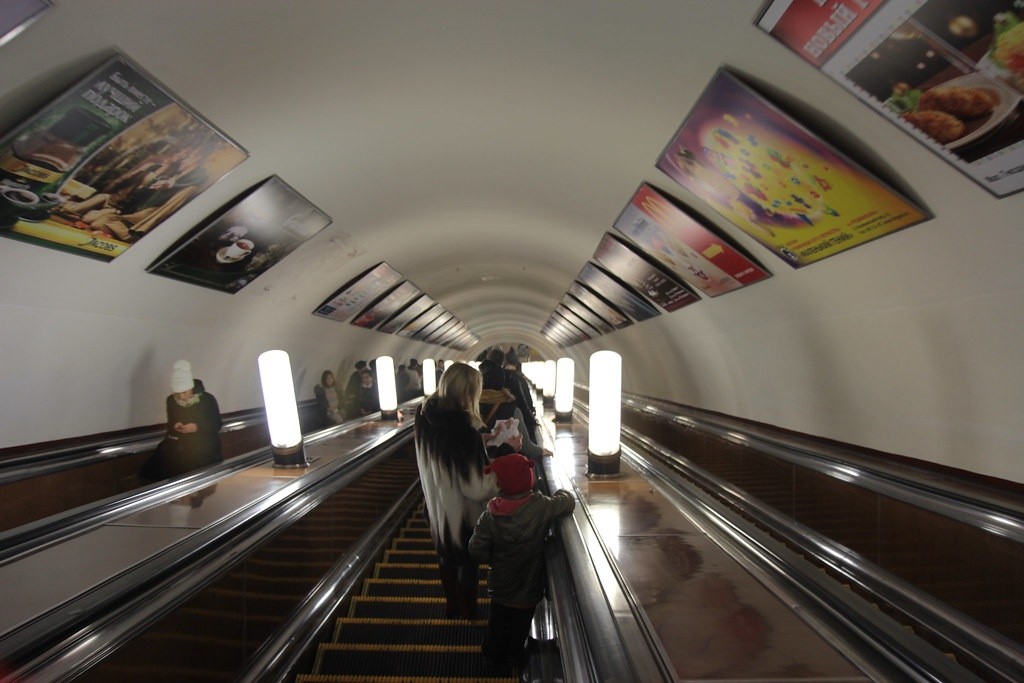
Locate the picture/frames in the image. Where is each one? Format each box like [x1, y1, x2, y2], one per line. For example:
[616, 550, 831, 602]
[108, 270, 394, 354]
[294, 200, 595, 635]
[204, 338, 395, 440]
[0, 0, 481, 354]
[541, 0, 1024, 349]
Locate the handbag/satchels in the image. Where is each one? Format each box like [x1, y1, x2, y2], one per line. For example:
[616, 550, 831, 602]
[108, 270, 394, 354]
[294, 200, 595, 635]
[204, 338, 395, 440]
[148, 433, 189, 479]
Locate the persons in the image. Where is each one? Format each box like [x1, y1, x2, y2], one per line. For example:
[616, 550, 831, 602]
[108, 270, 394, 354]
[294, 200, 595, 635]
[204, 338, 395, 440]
[467, 454, 575, 678]
[62, 125, 214, 242]
[313, 370, 346, 425]
[166, 359, 224, 472]
[345, 343, 553, 619]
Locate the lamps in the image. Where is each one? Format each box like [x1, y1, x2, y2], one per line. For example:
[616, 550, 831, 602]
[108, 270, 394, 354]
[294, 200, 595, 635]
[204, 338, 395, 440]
[421, 359, 437, 399]
[586, 352, 623, 478]
[543, 359, 556, 408]
[535, 361, 543, 395]
[376, 356, 399, 421]
[553, 358, 575, 422]
[257, 349, 311, 468]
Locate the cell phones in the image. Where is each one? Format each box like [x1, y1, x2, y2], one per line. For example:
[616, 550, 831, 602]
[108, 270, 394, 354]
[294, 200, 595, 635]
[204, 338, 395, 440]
[176, 426, 184, 430]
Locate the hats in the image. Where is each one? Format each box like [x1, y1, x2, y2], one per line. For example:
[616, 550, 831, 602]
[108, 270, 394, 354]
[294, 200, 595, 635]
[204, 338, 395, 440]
[479, 359, 505, 391]
[355, 361, 367, 368]
[483, 453, 537, 496]
